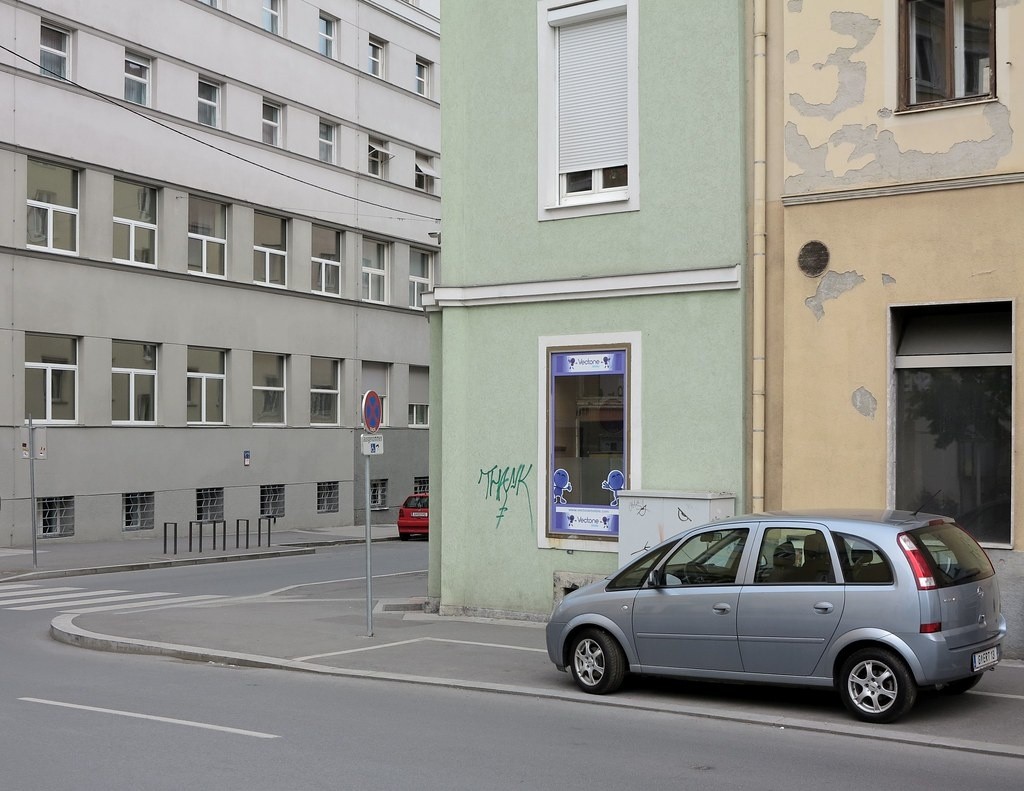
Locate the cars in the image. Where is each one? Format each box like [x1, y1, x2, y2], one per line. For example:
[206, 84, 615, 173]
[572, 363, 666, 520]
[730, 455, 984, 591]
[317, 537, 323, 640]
[541, 510, 1008, 723]
[396, 491, 430, 542]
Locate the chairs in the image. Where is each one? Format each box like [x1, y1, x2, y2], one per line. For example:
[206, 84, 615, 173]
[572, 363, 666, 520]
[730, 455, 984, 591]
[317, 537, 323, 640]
[762, 535, 891, 582]
